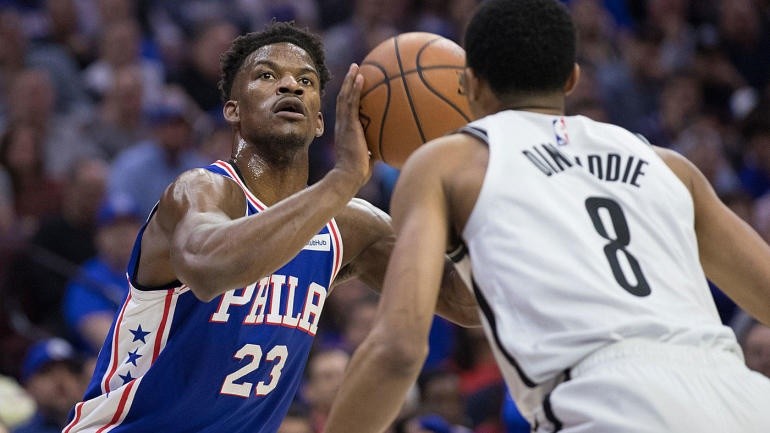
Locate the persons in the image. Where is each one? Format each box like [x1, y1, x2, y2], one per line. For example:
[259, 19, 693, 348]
[318, 1, 770, 433]
[0, 0, 770, 433]
[56, 21, 485, 433]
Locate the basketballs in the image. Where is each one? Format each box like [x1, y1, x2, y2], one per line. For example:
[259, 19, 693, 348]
[355, 28, 478, 169]
